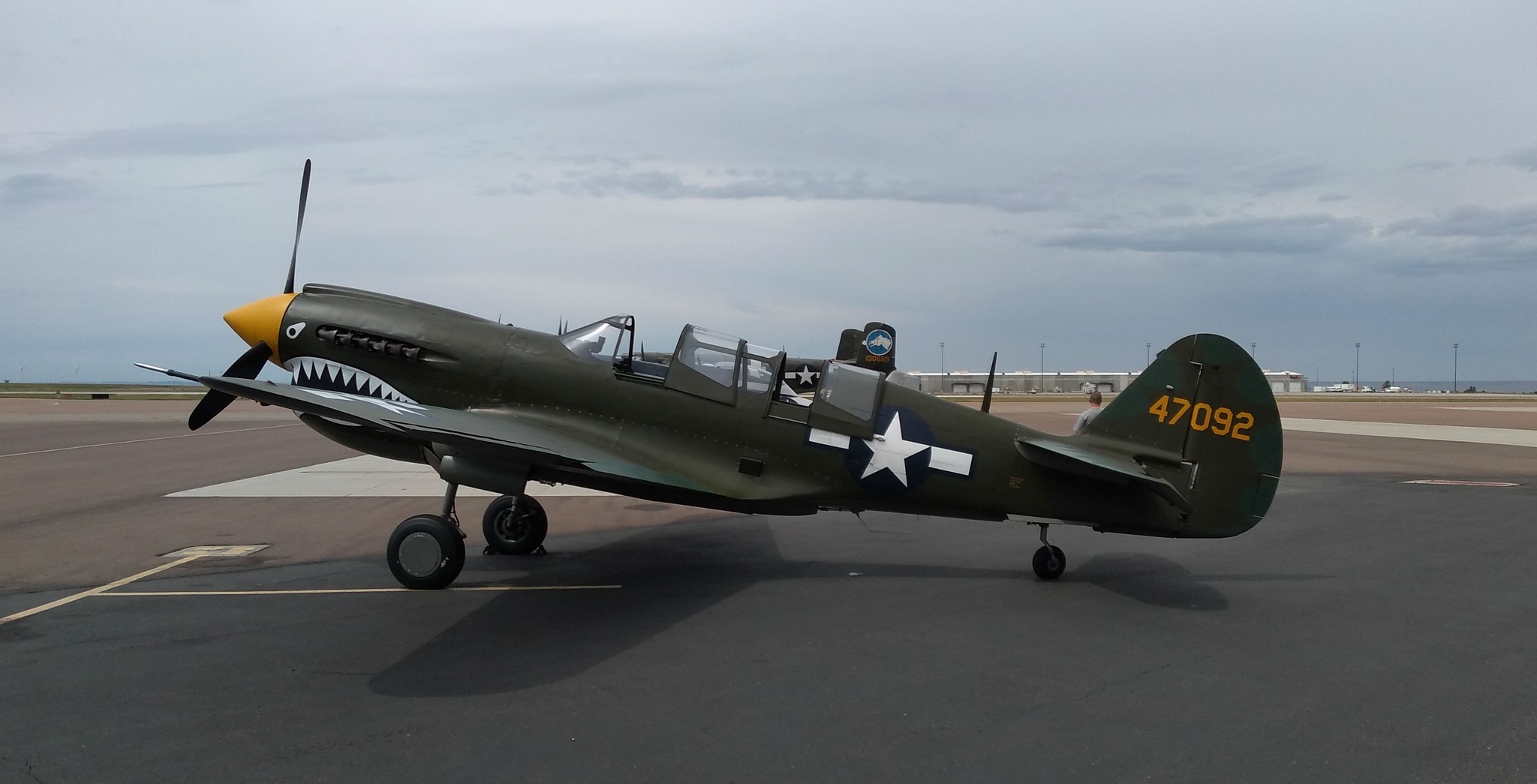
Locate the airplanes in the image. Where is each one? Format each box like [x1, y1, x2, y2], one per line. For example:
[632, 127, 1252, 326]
[133, 158, 1285, 591]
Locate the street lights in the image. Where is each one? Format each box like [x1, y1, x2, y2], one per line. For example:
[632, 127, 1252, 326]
[940, 342, 945, 393]
[1352, 371, 1355, 384]
[21, 367, 23, 383]
[1453, 343, 1459, 393]
[1040, 343, 1045, 393]
[76, 367, 78, 383]
[1355, 342, 1361, 393]
[1392, 368, 1395, 387]
[1146, 343, 1151, 367]
[1317, 368, 1319, 386]
[1252, 342, 1256, 358]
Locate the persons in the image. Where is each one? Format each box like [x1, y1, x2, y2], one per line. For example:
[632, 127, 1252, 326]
[1074, 390, 1105, 438]
